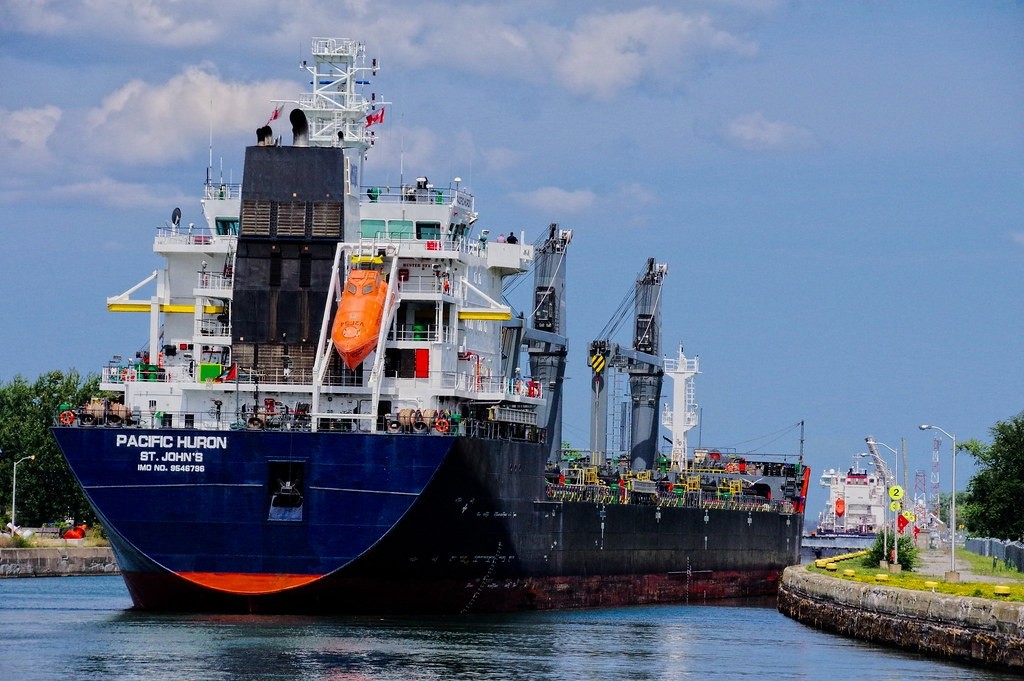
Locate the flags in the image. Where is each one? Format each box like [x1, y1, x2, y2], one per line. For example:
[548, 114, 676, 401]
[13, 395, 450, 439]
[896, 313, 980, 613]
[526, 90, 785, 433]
[365, 106, 384, 127]
[266, 105, 283, 126]
[213, 364, 237, 384]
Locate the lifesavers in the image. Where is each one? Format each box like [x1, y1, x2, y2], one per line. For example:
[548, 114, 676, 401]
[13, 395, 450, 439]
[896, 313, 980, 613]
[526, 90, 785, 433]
[444, 279, 448, 292]
[60, 411, 74, 424]
[413, 421, 428, 432]
[436, 420, 449, 432]
[892, 550, 894, 561]
[167, 373, 171, 383]
[248, 417, 262, 429]
[122, 370, 135, 381]
[727, 463, 735, 473]
[388, 421, 402, 433]
[107, 415, 122, 426]
[81, 414, 95, 425]
[516, 380, 520, 391]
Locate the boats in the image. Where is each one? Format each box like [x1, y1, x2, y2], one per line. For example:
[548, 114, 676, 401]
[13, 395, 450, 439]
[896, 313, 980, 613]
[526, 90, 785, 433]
[801, 454, 886, 559]
[49, 37, 810, 625]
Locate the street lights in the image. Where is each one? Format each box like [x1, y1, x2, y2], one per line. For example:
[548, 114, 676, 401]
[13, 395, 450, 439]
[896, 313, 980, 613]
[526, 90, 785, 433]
[860, 441, 899, 565]
[11, 455, 35, 538]
[919, 424, 956, 573]
